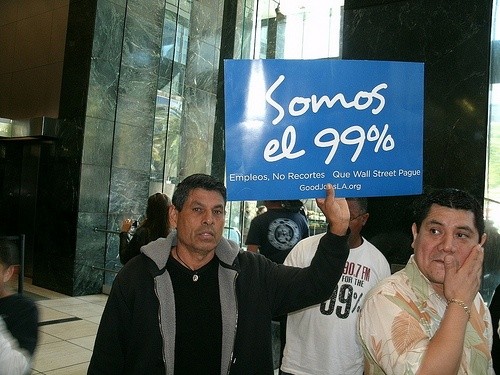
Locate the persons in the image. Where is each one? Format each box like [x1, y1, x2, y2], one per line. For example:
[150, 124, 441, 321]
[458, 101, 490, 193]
[0, 228, 40, 362]
[357, 189, 494, 375]
[118, 191, 172, 267]
[278, 198, 392, 375]
[243, 199, 313, 266]
[86, 172, 351, 375]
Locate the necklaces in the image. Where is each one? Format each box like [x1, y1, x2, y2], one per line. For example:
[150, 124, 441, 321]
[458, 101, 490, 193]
[175, 245, 202, 282]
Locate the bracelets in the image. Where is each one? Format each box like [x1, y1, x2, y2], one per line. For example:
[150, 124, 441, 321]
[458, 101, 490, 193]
[445, 297, 472, 320]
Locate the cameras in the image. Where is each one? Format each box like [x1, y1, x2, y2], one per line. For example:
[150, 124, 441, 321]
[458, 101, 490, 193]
[131, 220, 139, 227]
